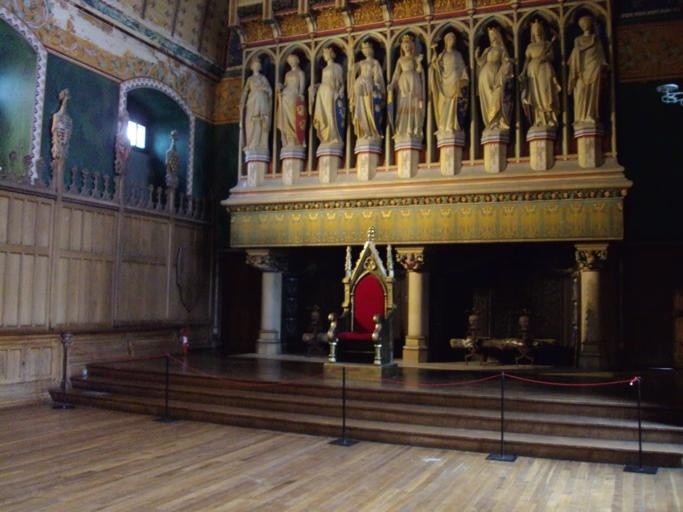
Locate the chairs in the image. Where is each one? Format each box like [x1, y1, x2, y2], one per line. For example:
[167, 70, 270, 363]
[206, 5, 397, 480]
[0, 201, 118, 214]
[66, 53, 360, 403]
[327, 226, 400, 367]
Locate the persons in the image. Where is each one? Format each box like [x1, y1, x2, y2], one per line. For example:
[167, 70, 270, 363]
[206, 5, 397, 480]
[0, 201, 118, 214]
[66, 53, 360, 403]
[238, 56, 272, 152]
[473, 25, 515, 130]
[517, 17, 563, 128]
[308, 46, 346, 147]
[566, 14, 609, 124]
[429, 30, 471, 135]
[274, 54, 306, 148]
[386, 33, 426, 140]
[349, 40, 386, 143]
